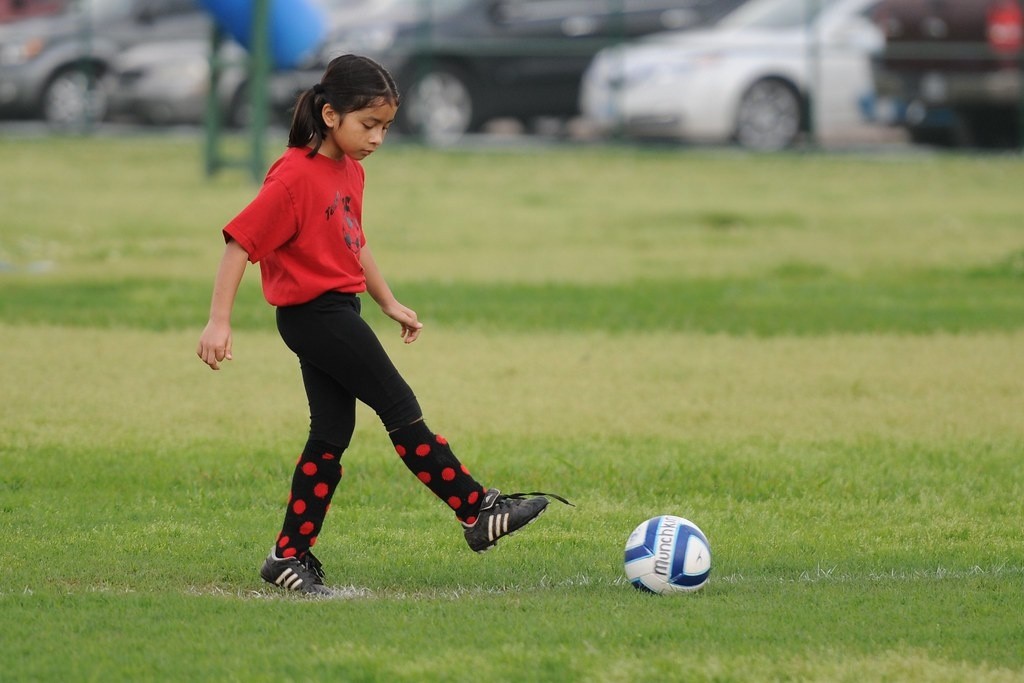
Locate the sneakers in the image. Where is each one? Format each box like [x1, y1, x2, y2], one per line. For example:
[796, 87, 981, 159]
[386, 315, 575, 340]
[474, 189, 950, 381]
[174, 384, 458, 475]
[258, 545, 333, 597]
[458, 488, 576, 554]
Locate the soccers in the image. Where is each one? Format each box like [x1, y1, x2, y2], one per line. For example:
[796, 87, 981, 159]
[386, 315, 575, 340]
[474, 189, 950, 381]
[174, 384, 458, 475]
[624, 513, 712, 602]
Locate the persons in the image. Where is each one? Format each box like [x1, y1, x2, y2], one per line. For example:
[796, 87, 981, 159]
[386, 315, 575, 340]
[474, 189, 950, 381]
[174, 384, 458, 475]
[195, 54, 550, 594]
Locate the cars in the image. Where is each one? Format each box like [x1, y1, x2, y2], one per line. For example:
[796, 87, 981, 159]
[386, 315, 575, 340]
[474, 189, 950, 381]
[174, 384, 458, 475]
[0, 2, 145, 134]
[579, 1, 906, 152]
[83, 14, 251, 132]
[862, 0, 1023, 149]
[383, 1, 741, 146]
